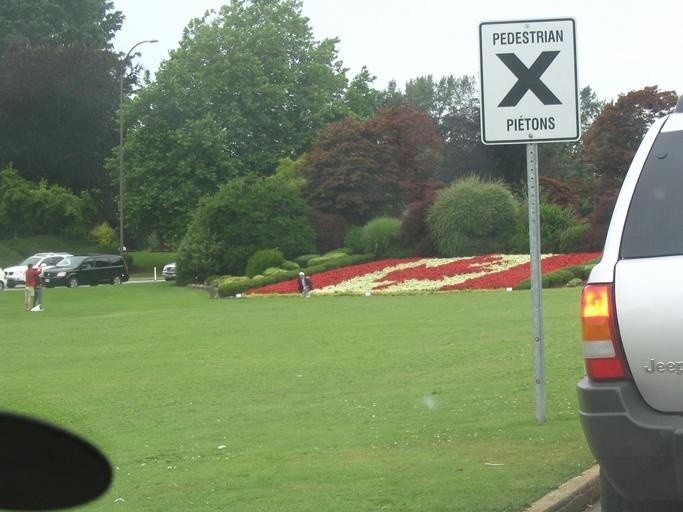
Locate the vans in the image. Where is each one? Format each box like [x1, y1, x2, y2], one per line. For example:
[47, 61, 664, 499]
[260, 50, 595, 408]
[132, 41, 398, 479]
[42, 254, 130, 288]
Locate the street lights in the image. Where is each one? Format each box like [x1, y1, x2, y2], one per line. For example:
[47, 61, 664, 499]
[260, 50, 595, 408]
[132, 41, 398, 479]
[118, 40, 160, 253]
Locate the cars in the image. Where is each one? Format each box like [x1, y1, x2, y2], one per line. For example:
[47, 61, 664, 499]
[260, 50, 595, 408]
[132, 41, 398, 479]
[162, 263, 178, 281]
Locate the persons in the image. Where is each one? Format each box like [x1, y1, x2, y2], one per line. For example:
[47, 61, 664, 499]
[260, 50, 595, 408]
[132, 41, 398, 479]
[297, 272, 312, 296]
[33, 269, 41, 309]
[24, 264, 42, 311]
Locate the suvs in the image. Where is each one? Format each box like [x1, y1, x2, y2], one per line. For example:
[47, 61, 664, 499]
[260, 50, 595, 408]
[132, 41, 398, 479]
[576, 95, 683, 512]
[3, 252, 75, 288]
